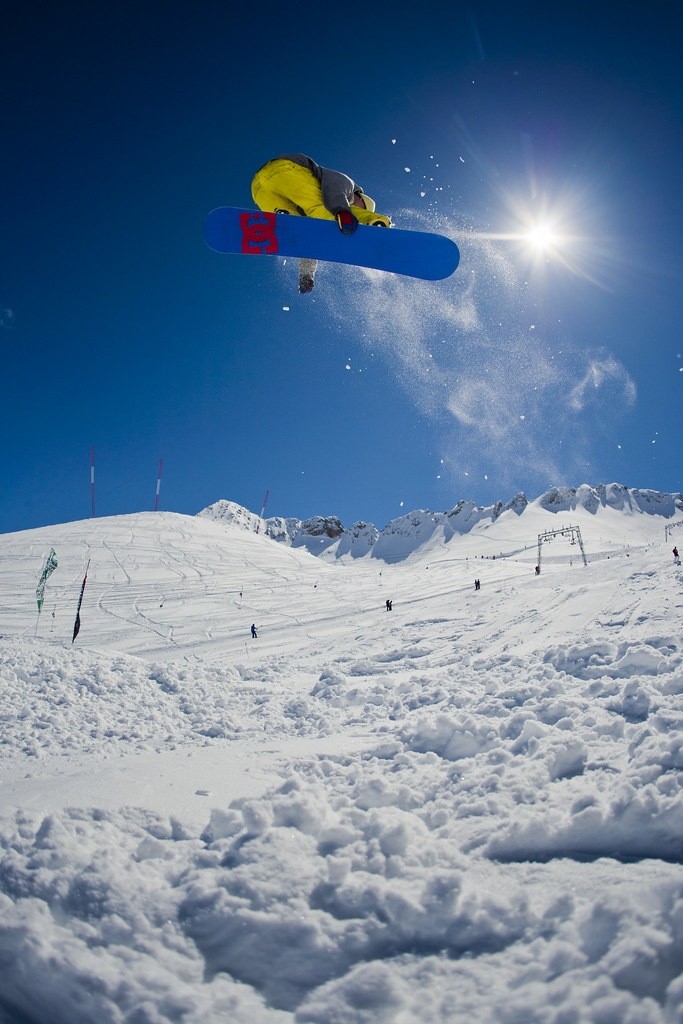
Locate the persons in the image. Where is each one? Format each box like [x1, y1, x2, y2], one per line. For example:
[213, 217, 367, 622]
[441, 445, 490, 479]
[389, 601, 392, 611]
[251, 624, 258, 638]
[535, 566, 539, 575]
[672, 546, 680, 562]
[475, 579, 478, 590]
[386, 600, 390, 611]
[481, 556, 483, 559]
[477, 579, 480, 589]
[251, 154, 392, 295]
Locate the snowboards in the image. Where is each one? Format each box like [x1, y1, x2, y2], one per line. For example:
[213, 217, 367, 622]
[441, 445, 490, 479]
[200, 205, 462, 282]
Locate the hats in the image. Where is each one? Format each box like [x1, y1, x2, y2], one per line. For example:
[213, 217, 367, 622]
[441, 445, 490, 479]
[360, 193, 375, 213]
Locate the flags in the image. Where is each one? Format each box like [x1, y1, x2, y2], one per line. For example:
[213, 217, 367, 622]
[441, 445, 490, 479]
[72, 570, 87, 643]
[36, 548, 58, 613]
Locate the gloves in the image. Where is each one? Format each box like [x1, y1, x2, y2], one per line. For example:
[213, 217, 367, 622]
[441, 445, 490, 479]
[335, 210, 358, 233]
[298, 276, 315, 293]
